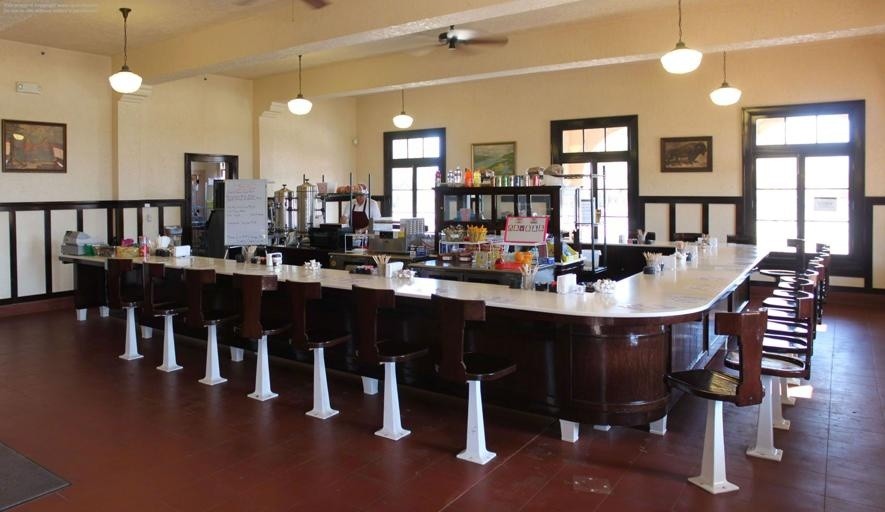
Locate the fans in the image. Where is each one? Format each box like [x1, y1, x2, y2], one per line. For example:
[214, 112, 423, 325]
[401, 25, 508, 59]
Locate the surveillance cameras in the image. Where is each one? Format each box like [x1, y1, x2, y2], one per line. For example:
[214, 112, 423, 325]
[439, 25, 458, 49]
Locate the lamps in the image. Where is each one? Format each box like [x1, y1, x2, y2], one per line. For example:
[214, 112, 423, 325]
[287, 55, 313, 116]
[659, 0, 704, 75]
[107, 8, 144, 96]
[392, 89, 414, 129]
[708, 51, 742, 107]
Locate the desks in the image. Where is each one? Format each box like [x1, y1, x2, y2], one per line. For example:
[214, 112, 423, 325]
[57, 229, 774, 435]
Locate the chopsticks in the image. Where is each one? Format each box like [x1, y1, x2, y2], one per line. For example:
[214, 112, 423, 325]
[676, 240, 687, 260]
[644, 251, 662, 272]
[243, 244, 258, 261]
[637, 229, 649, 242]
[518, 263, 538, 290]
[373, 255, 391, 277]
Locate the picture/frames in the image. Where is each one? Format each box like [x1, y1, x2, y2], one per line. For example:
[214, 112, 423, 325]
[659, 136, 713, 172]
[1, 118, 69, 175]
[470, 141, 516, 175]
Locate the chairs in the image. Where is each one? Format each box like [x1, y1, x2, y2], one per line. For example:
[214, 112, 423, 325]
[632, 231, 656, 240]
[352, 284, 429, 442]
[766, 268, 820, 407]
[759, 263, 825, 388]
[285, 278, 354, 420]
[736, 276, 816, 434]
[779, 243, 830, 280]
[771, 252, 832, 298]
[141, 260, 190, 374]
[778, 245, 833, 290]
[763, 255, 826, 307]
[672, 232, 701, 242]
[663, 307, 770, 495]
[430, 294, 519, 466]
[106, 257, 146, 359]
[727, 235, 748, 243]
[184, 268, 243, 386]
[234, 271, 290, 401]
[724, 290, 812, 464]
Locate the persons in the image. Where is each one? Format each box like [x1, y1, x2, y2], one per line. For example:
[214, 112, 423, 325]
[340, 184, 381, 236]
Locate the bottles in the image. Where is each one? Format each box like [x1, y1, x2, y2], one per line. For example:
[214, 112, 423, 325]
[465, 169, 472, 187]
[473, 169, 482, 187]
[533, 174, 541, 186]
[139, 236, 150, 262]
[455, 167, 462, 187]
[435, 170, 441, 188]
[447, 171, 454, 187]
[492, 174, 530, 187]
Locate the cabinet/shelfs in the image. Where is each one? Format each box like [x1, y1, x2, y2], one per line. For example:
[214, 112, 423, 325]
[408, 257, 555, 290]
[433, 186, 585, 292]
[329, 251, 411, 278]
